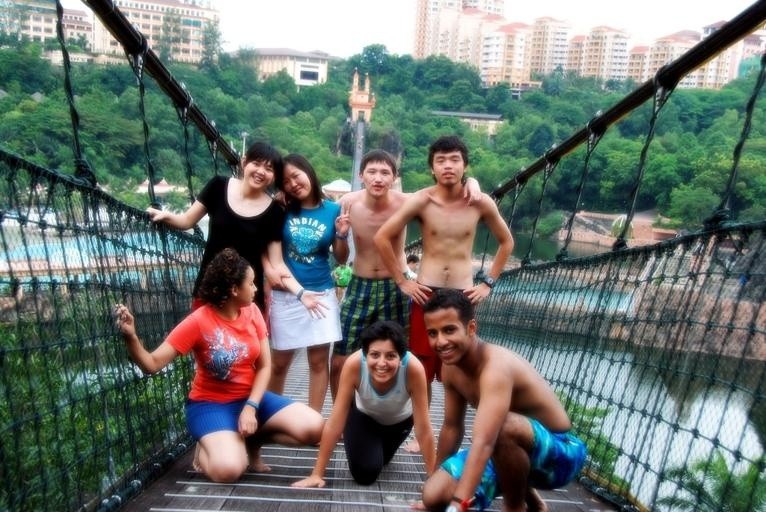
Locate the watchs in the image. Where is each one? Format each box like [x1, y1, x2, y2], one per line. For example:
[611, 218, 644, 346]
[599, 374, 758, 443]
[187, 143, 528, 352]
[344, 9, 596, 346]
[480, 274, 497, 289]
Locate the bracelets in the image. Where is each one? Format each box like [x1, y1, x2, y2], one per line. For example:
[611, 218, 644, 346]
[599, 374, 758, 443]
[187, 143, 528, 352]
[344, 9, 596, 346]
[245, 401, 260, 410]
[296, 288, 306, 300]
[451, 496, 476, 510]
[334, 232, 350, 240]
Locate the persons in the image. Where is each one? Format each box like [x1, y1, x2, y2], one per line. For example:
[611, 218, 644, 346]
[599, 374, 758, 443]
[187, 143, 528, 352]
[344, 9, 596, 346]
[114, 248, 326, 484]
[290, 320, 437, 487]
[409, 289, 587, 512]
[372, 136, 516, 455]
[330, 262, 352, 305]
[406, 254, 419, 278]
[271, 149, 483, 442]
[144, 142, 330, 333]
[260, 152, 353, 447]
